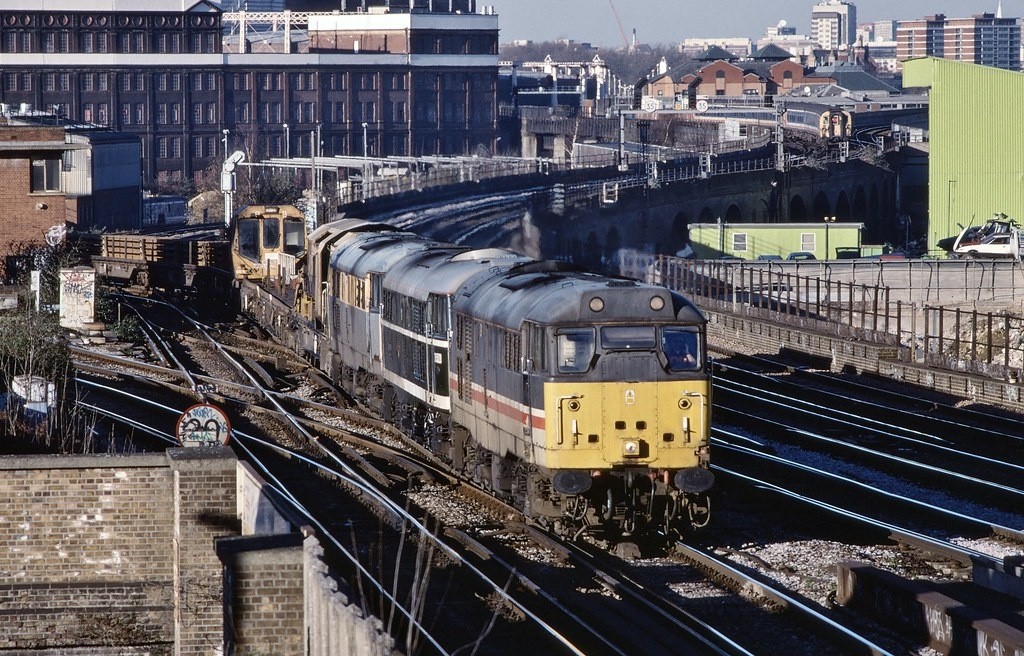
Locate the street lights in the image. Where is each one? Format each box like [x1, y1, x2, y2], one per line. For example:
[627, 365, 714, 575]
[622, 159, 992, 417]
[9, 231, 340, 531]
[221, 129, 231, 159]
[283, 123, 290, 159]
[493, 137, 501, 155]
[824, 216, 836, 260]
[362, 123, 368, 157]
[319, 141, 325, 190]
[947, 180, 957, 237]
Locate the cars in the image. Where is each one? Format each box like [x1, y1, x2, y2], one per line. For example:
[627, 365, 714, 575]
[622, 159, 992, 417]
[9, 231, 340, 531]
[786, 252, 818, 260]
[937, 213, 1024, 260]
[757, 255, 778, 260]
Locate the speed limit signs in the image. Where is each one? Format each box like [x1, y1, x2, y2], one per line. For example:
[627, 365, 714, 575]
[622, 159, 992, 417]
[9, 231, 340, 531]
[178, 403, 230, 451]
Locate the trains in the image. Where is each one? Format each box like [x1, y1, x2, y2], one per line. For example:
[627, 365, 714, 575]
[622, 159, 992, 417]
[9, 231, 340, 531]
[231, 206, 716, 543]
